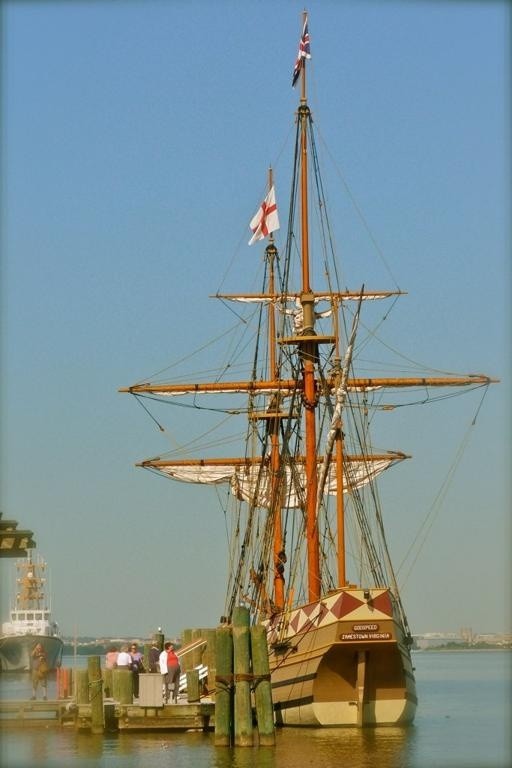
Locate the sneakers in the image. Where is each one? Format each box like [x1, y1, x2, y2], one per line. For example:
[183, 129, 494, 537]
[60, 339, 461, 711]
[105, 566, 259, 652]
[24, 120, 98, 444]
[29, 696, 47, 702]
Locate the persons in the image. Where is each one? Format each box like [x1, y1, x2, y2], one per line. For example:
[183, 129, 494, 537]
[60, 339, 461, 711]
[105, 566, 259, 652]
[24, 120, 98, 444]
[129, 643, 144, 699]
[117, 644, 132, 669]
[29, 641, 48, 701]
[148, 638, 162, 672]
[158, 642, 181, 704]
[104, 645, 119, 667]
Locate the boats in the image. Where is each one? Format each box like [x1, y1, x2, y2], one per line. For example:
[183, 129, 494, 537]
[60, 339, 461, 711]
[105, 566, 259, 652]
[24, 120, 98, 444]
[0, 548, 64, 673]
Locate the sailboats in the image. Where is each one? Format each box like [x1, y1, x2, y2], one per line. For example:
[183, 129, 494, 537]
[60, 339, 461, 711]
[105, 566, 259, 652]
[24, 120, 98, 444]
[118, 7, 502, 727]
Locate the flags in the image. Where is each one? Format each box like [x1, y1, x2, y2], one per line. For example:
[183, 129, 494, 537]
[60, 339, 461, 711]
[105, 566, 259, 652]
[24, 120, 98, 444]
[290, 12, 312, 89]
[246, 183, 280, 247]
